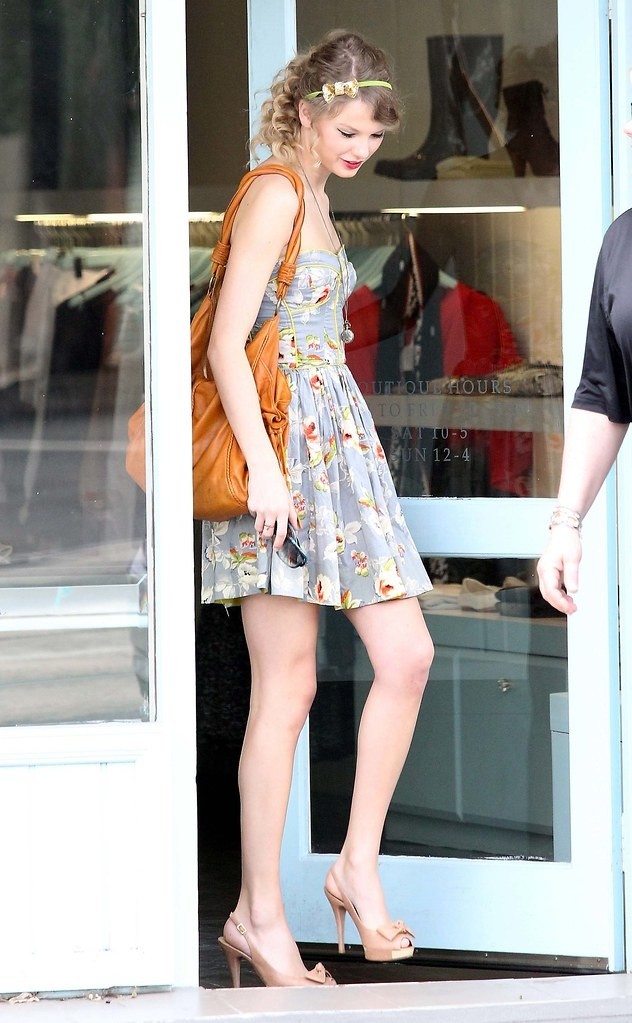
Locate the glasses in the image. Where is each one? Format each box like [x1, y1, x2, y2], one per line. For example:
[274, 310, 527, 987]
[274, 523, 307, 568]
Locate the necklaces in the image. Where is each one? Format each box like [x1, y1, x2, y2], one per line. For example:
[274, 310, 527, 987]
[294, 151, 355, 343]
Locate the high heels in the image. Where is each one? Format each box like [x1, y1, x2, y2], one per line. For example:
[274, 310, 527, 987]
[324, 867, 416, 962]
[218, 911, 337, 988]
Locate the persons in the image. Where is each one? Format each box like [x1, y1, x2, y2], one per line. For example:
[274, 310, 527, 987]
[533, 208, 632, 615]
[197, 31, 435, 988]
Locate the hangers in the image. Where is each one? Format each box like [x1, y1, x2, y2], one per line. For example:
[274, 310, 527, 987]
[1, 214, 457, 291]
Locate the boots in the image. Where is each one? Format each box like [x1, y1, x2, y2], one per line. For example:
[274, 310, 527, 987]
[502, 81, 560, 177]
[375, 38, 504, 180]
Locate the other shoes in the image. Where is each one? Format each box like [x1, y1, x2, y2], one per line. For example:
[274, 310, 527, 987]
[460, 578, 498, 612]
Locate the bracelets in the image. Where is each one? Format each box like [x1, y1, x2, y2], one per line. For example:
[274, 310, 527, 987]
[263, 524, 276, 531]
[549, 506, 582, 535]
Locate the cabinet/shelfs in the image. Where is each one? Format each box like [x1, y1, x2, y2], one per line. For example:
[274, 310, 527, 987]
[353, 579, 568, 858]
[1, 177, 564, 433]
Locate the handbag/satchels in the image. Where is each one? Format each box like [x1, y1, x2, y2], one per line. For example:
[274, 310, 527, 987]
[122, 164, 305, 520]
